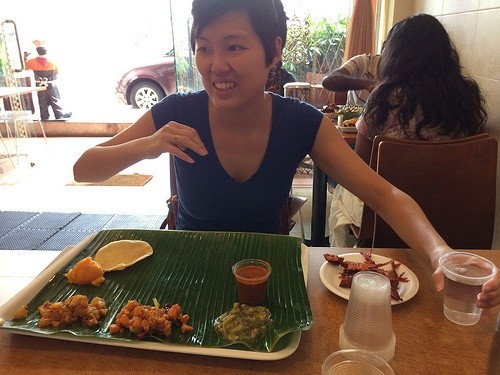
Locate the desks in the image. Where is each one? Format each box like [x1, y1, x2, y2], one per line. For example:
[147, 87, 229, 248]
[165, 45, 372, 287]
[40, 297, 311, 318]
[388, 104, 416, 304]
[0, 87, 48, 168]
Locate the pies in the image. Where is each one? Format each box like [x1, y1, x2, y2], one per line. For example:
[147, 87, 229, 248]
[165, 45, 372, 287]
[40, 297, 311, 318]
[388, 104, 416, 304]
[95, 239, 153, 271]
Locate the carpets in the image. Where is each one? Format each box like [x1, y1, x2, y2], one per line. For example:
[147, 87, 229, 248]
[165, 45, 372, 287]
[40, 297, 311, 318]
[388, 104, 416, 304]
[66, 174, 152, 186]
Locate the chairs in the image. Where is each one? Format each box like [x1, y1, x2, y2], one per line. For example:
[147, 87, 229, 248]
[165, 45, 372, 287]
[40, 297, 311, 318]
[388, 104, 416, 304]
[168, 153, 289, 235]
[351, 132, 498, 250]
[0, 71, 48, 164]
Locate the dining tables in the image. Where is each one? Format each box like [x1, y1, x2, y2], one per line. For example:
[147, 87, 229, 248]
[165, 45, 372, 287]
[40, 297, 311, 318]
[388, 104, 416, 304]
[302, 119, 358, 247]
[0, 247, 500, 375]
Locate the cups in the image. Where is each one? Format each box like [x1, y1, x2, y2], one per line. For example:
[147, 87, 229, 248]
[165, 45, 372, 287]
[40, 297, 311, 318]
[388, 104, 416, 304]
[338, 271, 396, 367]
[232, 260, 272, 305]
[322, 349, 396, 375]
[439, 252, 497, 326]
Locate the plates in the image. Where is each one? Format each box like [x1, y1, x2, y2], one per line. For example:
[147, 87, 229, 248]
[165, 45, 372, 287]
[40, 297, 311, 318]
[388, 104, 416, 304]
[340, 127, 358, 132]
[319, 253, 419, 305]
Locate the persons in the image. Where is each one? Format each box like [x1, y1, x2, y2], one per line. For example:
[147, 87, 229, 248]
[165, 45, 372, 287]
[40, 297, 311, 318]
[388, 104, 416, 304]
[73, 0, 500, 321]
[24, 39, 72, 120]
[322, 40, 386, 105]
[328, 13, 489, 247]
